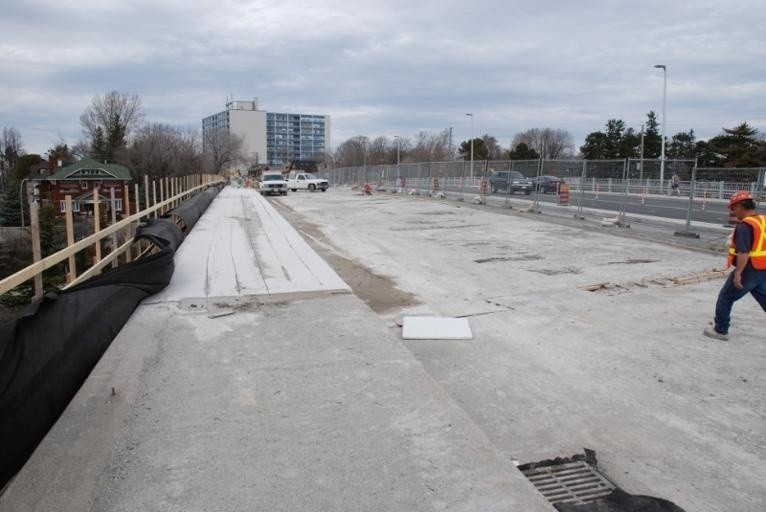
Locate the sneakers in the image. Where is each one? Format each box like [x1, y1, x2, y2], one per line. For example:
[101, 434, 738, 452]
[703, 329, 729, 341]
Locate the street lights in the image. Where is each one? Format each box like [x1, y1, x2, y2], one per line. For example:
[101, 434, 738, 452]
[394, 136, 400, 187]
[19, 177, 41, 228]
[652, 64, 667, 195]
[464, 113, 474, 179]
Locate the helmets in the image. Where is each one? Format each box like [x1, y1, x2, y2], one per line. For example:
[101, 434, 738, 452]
[727, 190, 753, 208]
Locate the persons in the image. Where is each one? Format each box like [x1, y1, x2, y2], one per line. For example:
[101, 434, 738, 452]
[703, 190, 766, 341]
[671, 172, 681, 197]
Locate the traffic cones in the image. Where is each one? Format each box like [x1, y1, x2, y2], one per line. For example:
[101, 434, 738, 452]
[724, 206, 740, 228]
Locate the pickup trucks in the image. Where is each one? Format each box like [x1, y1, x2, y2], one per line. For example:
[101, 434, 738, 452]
[283, 170, 331, 194]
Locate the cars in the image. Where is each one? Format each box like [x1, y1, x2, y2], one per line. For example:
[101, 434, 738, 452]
[528, 174, 567, 195]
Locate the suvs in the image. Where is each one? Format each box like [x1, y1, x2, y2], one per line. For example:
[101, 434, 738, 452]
[488, 170, 535, 197]
[257, 170, 290, 197]
